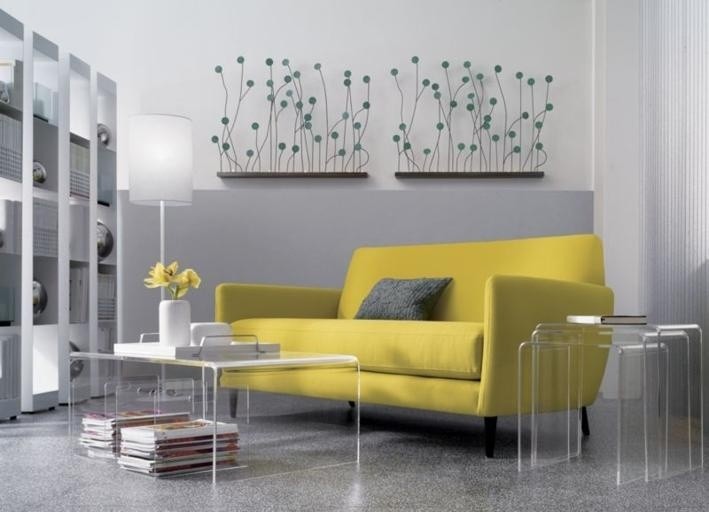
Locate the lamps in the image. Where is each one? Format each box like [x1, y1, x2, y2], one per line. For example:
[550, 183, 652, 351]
[128, 113, 194, 403]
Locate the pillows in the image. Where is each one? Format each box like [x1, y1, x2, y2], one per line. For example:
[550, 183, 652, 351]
[354, 278, 452, 320]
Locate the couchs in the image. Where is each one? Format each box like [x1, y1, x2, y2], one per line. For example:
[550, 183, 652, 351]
[216, 234, 614, 459]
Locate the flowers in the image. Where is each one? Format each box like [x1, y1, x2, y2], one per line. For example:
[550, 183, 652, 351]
[145, 260, 202, 300]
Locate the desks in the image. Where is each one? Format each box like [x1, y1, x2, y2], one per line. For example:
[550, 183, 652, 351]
[517, 323, 703, 487]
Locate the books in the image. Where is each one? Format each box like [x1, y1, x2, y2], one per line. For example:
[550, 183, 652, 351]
[565, 314, 650, 327]
[78, 408, 240, 480]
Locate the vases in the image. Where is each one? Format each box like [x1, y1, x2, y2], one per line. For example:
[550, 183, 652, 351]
[159, 301, 191, 348]
